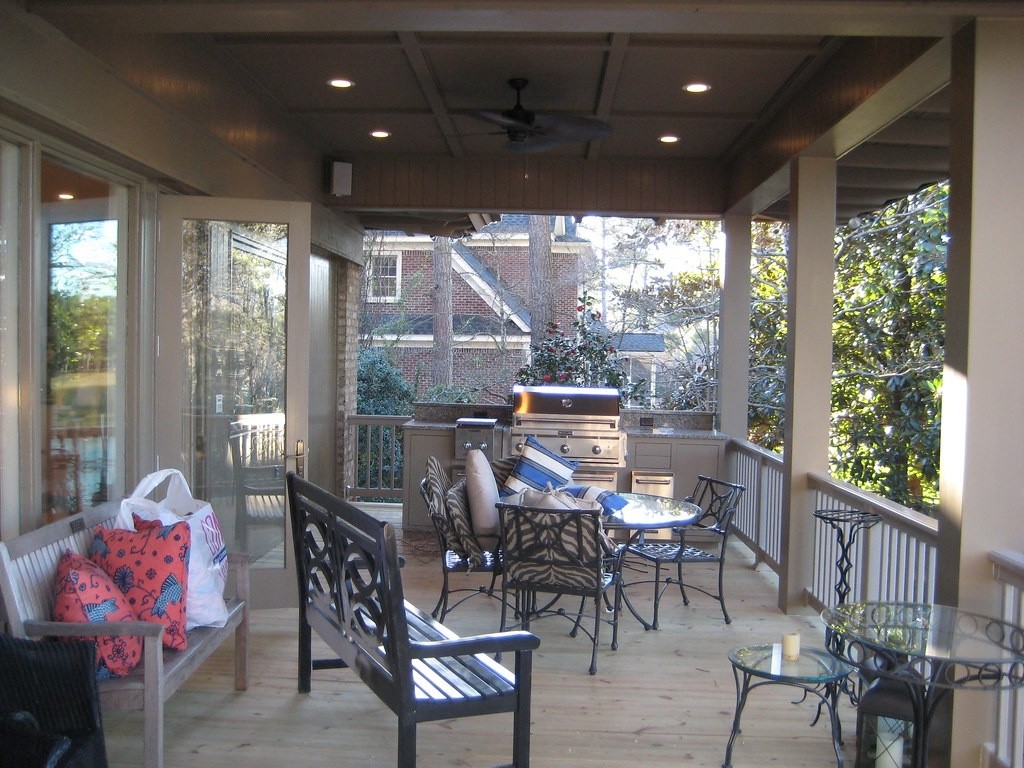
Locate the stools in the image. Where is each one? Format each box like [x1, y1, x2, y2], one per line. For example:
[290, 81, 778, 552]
[857, 668, 923, 768]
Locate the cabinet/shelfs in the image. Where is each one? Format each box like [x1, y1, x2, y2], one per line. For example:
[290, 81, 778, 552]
[630, 471, 675, 540]
[617, 437, 726, 541]
[403, 429, 503, 532]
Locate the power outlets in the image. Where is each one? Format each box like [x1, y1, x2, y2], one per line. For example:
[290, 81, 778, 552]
[640, 418, 653, 426]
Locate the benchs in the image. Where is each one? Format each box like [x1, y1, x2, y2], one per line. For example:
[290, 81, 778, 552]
[229, 422, 285, 554]
[286, 470, 540, 768]
[0, 494, 249, 767]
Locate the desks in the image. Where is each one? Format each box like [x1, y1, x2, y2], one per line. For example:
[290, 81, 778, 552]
[721, 644, 854, 768]
[821, 602, 1023, 768]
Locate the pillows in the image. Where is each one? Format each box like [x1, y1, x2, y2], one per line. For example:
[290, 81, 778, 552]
[53, 548, 143, 679]
[465, 449, 501, 553]
[89, 520, 190, 651]
[507, 481, 621, 553]
[500, 435, 578, 496]
[557, 485, 629, 515]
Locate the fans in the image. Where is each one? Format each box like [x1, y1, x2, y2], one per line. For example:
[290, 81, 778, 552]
[429, 78, 615, 155]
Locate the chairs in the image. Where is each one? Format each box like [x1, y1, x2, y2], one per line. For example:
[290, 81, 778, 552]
[420, 477, 747, 675]
[0, 632, 109, 768]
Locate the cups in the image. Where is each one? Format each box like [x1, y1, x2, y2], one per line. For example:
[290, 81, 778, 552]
[781, 632, 800, 661]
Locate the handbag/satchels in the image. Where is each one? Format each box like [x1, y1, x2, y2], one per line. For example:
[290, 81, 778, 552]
[113, 469, 228, 632]
